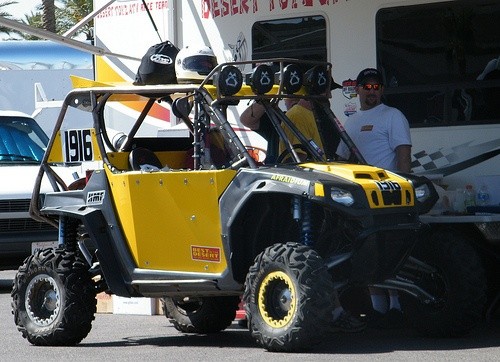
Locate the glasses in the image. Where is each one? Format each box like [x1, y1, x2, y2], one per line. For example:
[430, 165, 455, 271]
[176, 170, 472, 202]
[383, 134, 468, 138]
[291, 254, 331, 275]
[363, 83, 381, 91]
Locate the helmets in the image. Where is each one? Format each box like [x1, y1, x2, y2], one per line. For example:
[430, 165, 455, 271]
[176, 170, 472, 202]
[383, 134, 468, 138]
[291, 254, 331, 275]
[175, 43, 217, 83]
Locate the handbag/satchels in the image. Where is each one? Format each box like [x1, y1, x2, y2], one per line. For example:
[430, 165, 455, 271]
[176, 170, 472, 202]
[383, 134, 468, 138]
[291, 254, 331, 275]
[134, 40, 180, 85]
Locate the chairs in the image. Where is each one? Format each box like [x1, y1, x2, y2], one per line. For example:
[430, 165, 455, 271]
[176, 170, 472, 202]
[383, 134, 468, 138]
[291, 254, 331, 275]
[0, 125, 44, 161]
[129, 146, 163, 171]
[199, 143, 230, 169]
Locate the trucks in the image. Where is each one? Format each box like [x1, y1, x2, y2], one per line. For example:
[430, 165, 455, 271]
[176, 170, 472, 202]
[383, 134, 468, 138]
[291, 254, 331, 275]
[91, 1, 500, 273]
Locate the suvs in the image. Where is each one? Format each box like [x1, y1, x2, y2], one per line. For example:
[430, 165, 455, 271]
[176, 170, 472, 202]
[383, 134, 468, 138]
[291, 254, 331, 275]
[10, 55, 439, 350]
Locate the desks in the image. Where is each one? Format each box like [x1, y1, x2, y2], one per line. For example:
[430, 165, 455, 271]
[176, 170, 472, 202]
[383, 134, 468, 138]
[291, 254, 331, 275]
[420, 210, 500, 327]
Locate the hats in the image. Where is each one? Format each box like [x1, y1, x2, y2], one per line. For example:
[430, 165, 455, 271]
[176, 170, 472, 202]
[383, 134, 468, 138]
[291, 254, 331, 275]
[356, 68, 384, 86]
[307, 72, 343, 95]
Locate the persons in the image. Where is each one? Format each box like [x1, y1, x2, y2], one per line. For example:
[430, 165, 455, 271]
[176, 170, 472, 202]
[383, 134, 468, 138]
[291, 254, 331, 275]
[280, 68, 367, 333]
[240, 98, 298, 164]
[335, 68, 412, 329]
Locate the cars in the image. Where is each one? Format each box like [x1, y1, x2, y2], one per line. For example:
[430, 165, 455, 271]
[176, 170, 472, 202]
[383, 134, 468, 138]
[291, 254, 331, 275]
[0, 111, 59, 267]
